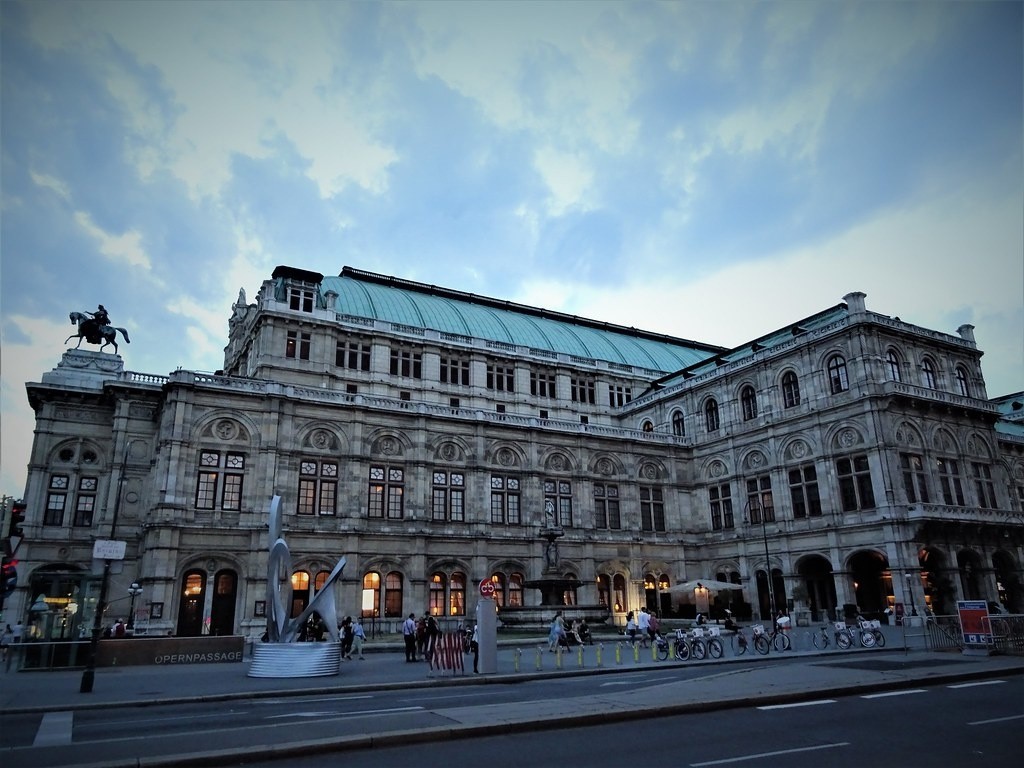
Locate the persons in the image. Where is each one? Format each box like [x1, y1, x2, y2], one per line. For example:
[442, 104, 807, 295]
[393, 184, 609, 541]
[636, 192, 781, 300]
[303, 613, 368, 661]
[104, 618, 126, 640]
[544, 498, 555, 519]
[695, 609, 787, 635]
[472, 624, 478, 674]
[628, 607, 662, 649]
[402, 611, 440, 661]
[0, 620, 24, 661]
[84, 305, 109, 326]
[548, 611, 594, 652]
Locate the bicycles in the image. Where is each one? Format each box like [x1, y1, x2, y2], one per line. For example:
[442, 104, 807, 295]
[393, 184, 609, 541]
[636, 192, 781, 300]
[463, 630, 473, 655]
[944, 613, 1023, 640]
[657, 628, 724, 661]
[812, 619, 885, 650]
[730, 623, 790, 655]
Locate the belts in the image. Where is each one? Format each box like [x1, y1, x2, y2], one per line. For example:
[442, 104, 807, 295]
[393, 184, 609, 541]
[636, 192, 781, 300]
[356, 635, 362, 637]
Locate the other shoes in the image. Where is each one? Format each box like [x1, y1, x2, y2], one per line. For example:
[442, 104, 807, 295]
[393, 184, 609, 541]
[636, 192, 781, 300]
[549, 650, 553, 652]
[412, 659, 418, 663]
[406, 659, 411, 663]
[347, 655, 352, 660]
[625, 641, 630, 646]
[359, 658, 365, 660]
[631, 644, 635, 648]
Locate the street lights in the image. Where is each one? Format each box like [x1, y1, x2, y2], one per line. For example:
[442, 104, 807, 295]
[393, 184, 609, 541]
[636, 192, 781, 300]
[743, 500, 779, 651]
[124, 583, 143, 639]
[78, 442, 132, 691]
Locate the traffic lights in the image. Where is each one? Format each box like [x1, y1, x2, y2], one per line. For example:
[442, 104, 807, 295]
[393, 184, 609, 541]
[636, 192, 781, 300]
[1, 556, 18, 576]
[1, 498, 27, 540]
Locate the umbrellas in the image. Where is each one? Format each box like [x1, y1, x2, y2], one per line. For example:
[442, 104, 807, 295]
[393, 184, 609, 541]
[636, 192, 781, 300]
[660, 578, 745, 619]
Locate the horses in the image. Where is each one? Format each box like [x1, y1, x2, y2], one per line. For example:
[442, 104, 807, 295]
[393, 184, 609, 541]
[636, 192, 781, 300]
[64, 312, 130, 354]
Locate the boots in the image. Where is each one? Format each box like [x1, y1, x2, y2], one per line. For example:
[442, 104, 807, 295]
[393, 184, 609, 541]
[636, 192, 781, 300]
[836, 622, 846, 631]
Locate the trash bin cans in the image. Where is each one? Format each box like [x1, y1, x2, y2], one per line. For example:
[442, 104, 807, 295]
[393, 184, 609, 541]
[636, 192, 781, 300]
[777, 617, 792, 650]
[843, 603, 860, 628]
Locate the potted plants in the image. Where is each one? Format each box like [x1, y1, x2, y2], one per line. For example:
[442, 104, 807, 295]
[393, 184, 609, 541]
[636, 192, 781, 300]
[791, 584, 808, 608]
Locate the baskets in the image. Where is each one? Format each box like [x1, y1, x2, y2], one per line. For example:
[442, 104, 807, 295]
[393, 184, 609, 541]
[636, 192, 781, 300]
[693, 628, 704, 638]
[753, 625, 765, 635]
[870, 621, 882, 630]
[709, 628, 720, 637]
[862, 621, 871, 630]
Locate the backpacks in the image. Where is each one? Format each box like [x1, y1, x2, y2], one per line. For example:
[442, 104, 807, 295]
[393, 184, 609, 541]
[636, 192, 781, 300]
[428, 616, 439, 631]
